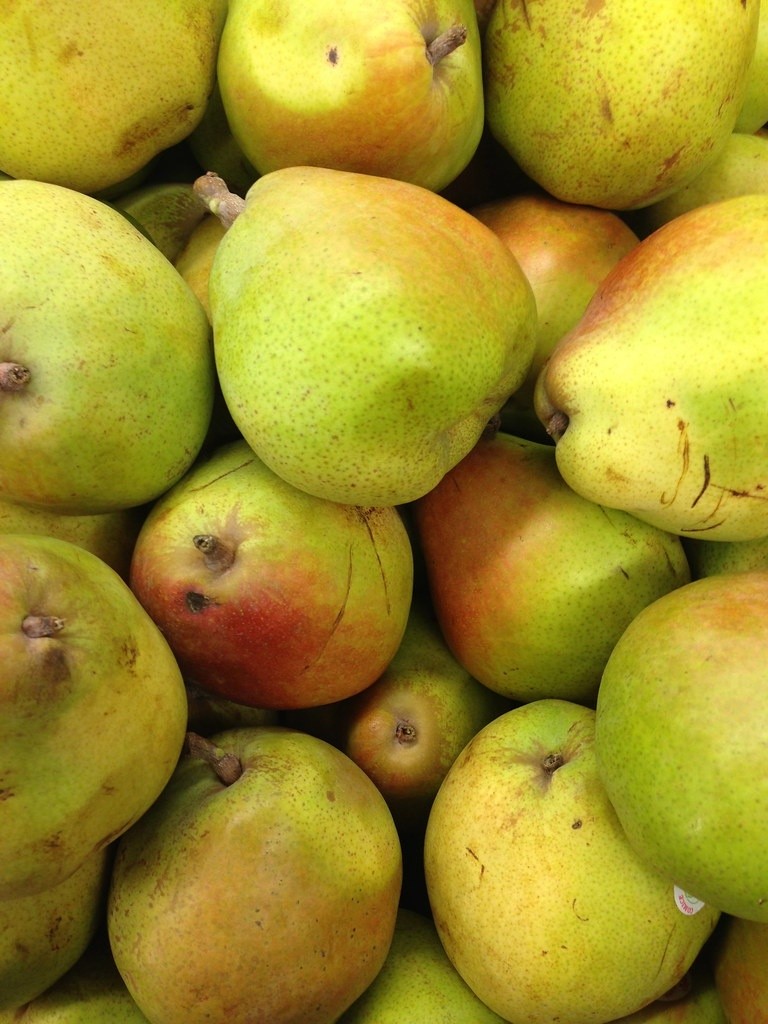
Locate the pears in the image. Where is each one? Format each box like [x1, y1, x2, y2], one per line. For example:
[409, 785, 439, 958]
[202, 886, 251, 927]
[0, 0, 768, 1024]
